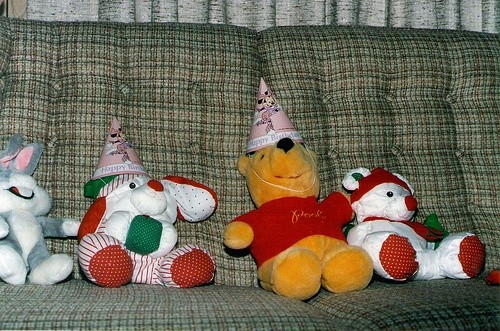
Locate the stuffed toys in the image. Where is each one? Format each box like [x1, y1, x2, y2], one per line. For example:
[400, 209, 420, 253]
[0, 134, 82, 286]
[224, 137, 374, 300]
[342, 168, 485, 281]
[77, 174, 218, 288]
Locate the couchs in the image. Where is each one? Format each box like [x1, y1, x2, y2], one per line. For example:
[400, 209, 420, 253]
[1, 15, 500, 331]
